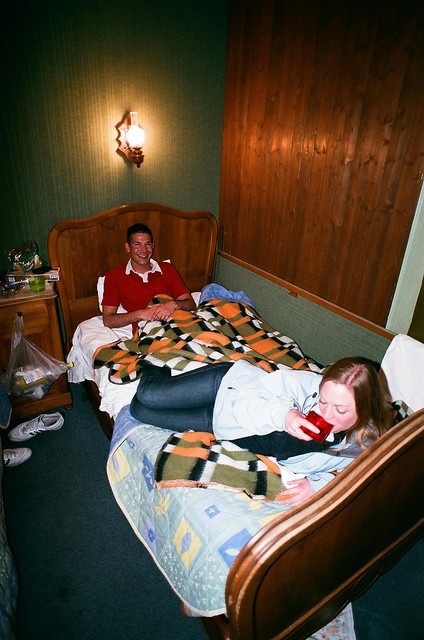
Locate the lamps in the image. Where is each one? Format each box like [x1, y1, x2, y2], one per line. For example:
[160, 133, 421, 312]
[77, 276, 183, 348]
[115, 110, 146, 168]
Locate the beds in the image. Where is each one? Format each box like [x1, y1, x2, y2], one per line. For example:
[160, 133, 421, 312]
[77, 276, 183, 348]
[47, 202, 424, 640]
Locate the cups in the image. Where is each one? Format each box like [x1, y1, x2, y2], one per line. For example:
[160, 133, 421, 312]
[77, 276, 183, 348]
[300, 410, 333, 443]
[29, 276, 45, 293]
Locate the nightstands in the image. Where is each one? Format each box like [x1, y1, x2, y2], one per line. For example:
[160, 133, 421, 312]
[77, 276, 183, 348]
[0, 281, 73, 420]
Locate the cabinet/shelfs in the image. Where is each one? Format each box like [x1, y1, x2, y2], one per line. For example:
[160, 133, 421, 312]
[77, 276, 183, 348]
[217, 0, 424, 330]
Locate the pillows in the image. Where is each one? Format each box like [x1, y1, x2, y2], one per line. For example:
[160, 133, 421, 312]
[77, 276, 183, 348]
[97, 259, 171, 314]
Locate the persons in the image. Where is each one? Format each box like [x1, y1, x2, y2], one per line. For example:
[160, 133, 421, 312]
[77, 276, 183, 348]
[100, 224, 196, 328]
[128, 356, 393, 509]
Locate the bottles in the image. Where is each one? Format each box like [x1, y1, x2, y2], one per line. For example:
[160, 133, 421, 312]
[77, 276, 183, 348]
[10, 362, 74, 390]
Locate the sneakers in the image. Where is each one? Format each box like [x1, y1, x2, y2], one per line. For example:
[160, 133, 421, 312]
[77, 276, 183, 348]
[8, 412, 65, 442]
[1, 448, 32, 467]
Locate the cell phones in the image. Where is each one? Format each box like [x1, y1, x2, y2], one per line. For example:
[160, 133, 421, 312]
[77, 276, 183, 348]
[32, 264, 53, 274]
